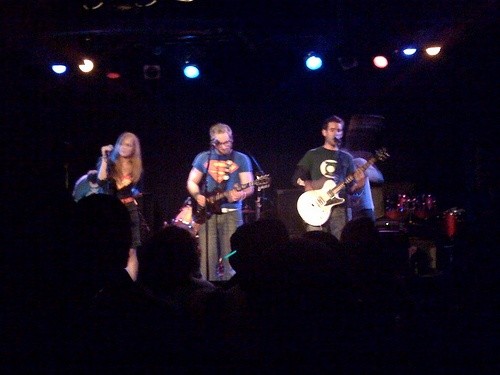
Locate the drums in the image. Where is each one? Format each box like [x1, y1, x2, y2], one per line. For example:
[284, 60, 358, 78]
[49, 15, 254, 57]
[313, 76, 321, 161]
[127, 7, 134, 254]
[442, 207, 467, 242]
[410, 238, 453, 276]
[408, 195, 443, 227]
[385, 191, 409, 219]
[173, 205, 201, 236]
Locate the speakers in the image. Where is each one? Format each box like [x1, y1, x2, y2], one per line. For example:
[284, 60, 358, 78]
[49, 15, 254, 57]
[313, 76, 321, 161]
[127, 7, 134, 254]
[276, 188, 309, 239]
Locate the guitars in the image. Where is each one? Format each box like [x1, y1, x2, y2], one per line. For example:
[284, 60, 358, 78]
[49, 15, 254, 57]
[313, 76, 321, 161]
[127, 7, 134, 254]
[192, 174, 270, 226]
[297, 149, 389, 227]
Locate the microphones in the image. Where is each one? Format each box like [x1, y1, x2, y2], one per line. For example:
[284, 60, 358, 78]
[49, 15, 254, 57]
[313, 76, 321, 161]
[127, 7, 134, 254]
[210, 138, 219, 148]
[106, 151, 111, 156]
[334, 136, 342, 144]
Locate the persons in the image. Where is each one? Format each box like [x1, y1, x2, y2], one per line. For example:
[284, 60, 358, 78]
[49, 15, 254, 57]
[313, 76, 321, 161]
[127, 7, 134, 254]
[0, 194, 500, 375]
[294, 116, 387, 240]
[187, 124, 255, 280]
[74, 132, 143, 283]
